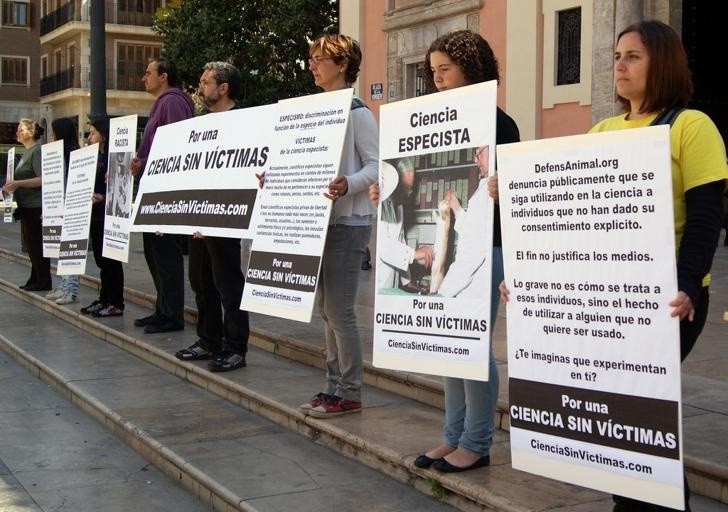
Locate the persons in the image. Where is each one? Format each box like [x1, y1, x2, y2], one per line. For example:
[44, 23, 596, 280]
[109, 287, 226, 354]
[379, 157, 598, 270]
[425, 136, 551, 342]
[45, 118, 81, 303]
[115, 162, 129, 217]
[381, 157, 433, 289]
[81, 120, 127, 317]
[154, 61, 249, 372]
[3, 118, 53, 291]
[440, 146, 488, 301]
[254, 35, 380, 420]
[369, 31, 520, 472]
[497, 20, 728, 512]
[105, 58, 194, 334]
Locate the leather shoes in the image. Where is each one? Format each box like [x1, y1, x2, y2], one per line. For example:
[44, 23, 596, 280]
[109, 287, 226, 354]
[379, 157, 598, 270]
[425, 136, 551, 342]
[434, 454, 489, 472]
[414, 454, 438, 469]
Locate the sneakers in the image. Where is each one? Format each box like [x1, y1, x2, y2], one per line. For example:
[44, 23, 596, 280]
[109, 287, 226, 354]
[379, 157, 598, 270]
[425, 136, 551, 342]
[135, 312, 184, 333]
[20, 283, 52, 291]
[80, 301, 124, 317]
[208, 351, 246, 372]
[299, 392, 362, 418]
[46, 290, 79, 305]
[175, 341, 223, 361]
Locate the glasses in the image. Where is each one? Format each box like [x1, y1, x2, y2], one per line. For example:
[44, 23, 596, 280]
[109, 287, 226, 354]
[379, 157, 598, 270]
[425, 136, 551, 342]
[308, 57, 332, 65]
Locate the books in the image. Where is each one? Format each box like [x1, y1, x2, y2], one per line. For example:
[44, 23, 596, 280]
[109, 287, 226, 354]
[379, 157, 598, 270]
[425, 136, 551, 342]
[406, 147, 485, 212]
